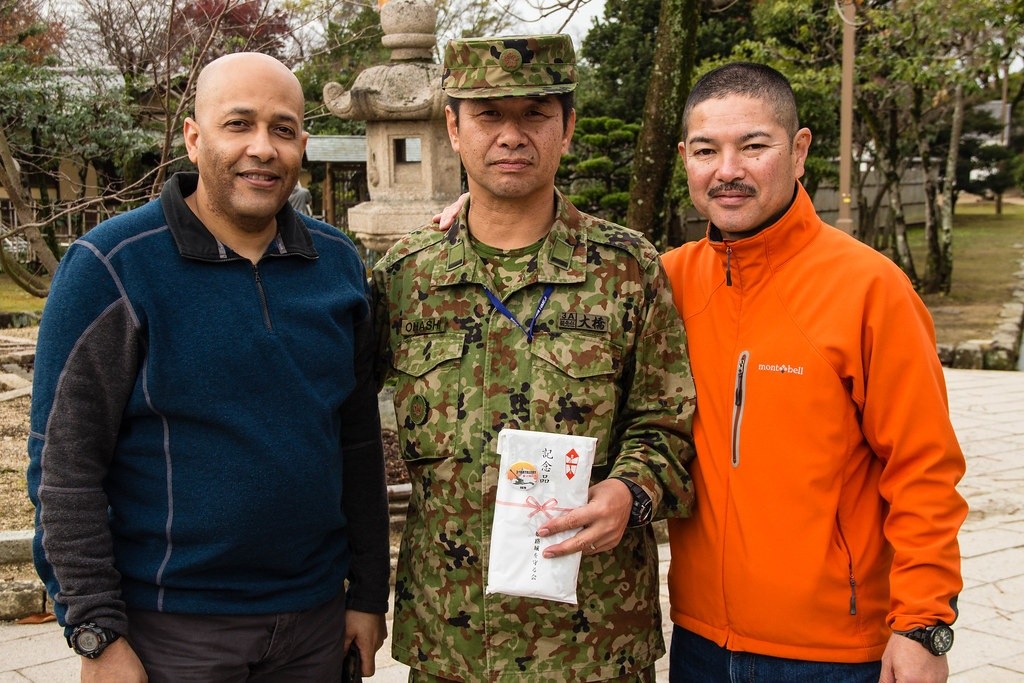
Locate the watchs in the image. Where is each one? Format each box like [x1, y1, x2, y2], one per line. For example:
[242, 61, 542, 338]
[902, 624, 954, 656]
[66, 623, 120, 660]
[617, 477, 653, 527]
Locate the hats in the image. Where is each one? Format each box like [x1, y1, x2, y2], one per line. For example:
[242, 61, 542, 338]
[442, 34, 580, 99]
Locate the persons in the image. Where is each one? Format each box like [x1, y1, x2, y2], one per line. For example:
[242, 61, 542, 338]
[369, 34, 697, 683]
[430, 62, 970, 683]
[287, 179, 313, 217]
[26, 52, 390, 683]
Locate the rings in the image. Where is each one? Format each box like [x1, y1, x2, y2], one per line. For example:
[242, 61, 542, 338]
[590, 544, 596, 551]
